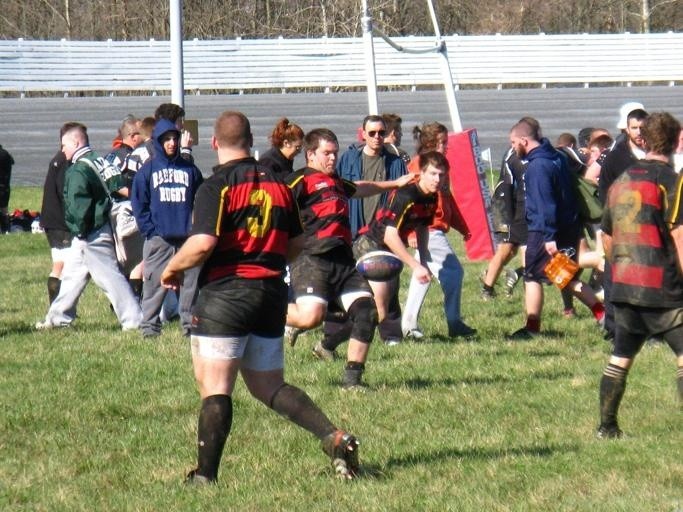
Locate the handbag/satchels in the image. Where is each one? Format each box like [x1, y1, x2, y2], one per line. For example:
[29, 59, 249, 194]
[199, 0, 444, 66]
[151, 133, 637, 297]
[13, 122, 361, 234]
[15, 215, 33, 232]
[107, 200, 146, 273]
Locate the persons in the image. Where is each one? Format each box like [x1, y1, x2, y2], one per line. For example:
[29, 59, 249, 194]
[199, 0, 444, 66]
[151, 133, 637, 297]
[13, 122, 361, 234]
[472, 102, 683, 438]
[30, 102, 479, 389]
[154, 111, 361, 488]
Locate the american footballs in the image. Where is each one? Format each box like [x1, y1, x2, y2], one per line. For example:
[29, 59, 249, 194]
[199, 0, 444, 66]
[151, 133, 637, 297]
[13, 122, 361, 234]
[355, 250, 404, 281]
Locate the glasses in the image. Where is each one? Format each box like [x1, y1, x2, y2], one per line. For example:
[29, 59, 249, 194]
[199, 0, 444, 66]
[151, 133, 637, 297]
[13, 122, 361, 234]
[368, 131, 386, 137]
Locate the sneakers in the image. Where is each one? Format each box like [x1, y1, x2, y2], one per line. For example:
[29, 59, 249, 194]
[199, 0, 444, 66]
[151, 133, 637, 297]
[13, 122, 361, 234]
[183, 469, 219, 485]
[508, 328, 535, 340]
[597, 426, 629, 439]
[563, 308, 576, 318]
[340, 382, 376, 395]
[331, 430, 359, 480]
[30, 318, 50, 330]
[597, 310, 606, 333]
[479, 268, 518, 300]
[286, 325, 476, 365]
